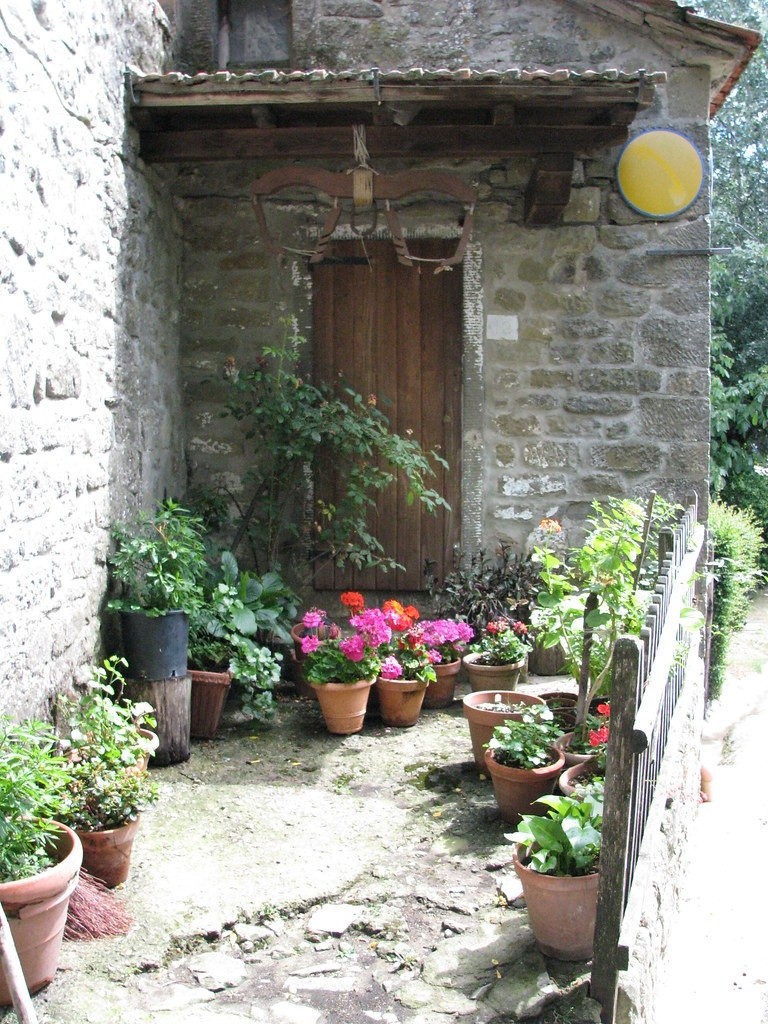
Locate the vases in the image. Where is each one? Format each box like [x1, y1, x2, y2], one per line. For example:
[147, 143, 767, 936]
[557, 759, 606, 799]
[377, 679, 430, 726]
[464, 653, 525, 692]
[291, 622, 335, 663]
[309, 676, 378, 738]
[423, 656, 461, 709]
[591, 696, 612, 722]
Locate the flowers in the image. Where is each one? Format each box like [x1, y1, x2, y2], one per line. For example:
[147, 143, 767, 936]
[573, 703, 610, 756]
[583, 721, 614, 787]
[468, 614, 537, 665]
[340, 591, 439, 688]
[311, 609, 342, 640]
[418, 617, 476, 666]
[301, 608, 393, 683]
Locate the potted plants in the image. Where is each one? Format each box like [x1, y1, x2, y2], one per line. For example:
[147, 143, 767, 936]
[0, 711, 85, 1012]
[502, 794, 604, 963]
[84, 653, 161, 773]
[463, 690, 547, 772]
[63, 747, 160, 888]
[538, 692, 578, 713]
[91, 506, 194, 682]
[483, 703, 567, 826]
[188, 581, 285, 740]
[554, 712, 576, 733]
[529, 495, 649, 768]
